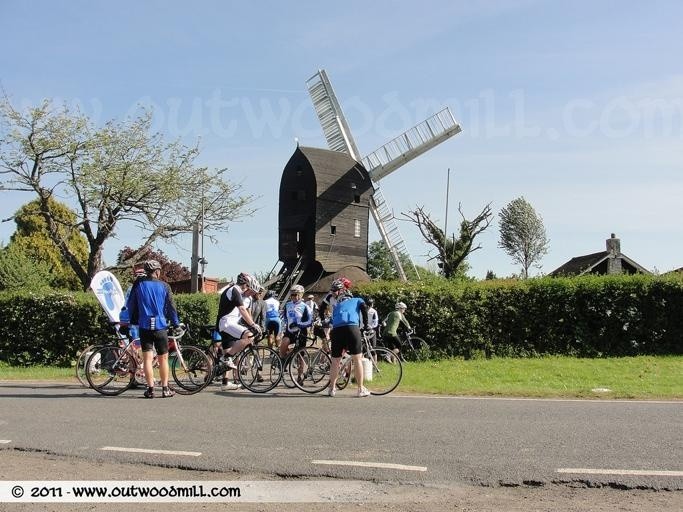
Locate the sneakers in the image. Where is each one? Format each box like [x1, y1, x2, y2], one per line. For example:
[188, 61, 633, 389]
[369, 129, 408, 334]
[162, 390, 175, 397]
[219, 354, 238, 391]
[143, 391, 154, 398]
[357, 386, 371, 397]
[383, 352, 393, 365]
[328, 387, 337, 396]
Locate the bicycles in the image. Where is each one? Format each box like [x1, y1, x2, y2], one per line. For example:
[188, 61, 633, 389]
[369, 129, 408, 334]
[75, 304, 402, 399]
[361, 324, 430, 366]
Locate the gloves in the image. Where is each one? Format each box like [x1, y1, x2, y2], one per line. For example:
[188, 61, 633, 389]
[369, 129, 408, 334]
[173, 326, 184, 336]
[249, 322, 263, 335]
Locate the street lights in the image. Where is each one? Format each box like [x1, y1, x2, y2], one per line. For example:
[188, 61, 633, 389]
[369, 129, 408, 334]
[189, 257, 208, 294]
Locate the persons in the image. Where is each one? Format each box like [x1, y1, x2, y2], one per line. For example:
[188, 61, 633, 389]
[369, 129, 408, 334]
[127, 260, 184, 398]
[204, 271, 411, 398]
[123, 269, 156, 390]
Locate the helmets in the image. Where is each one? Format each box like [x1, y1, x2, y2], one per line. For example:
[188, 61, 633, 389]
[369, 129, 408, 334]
[237, 272, 276, 297]
[394, 301, 408, 310]
[364, 296, 375, 304]
[289, 284, 305, 293]
[308, 295, 314, 299]
[331, 278, 353, 303]
[134, 259, 162, 277]
